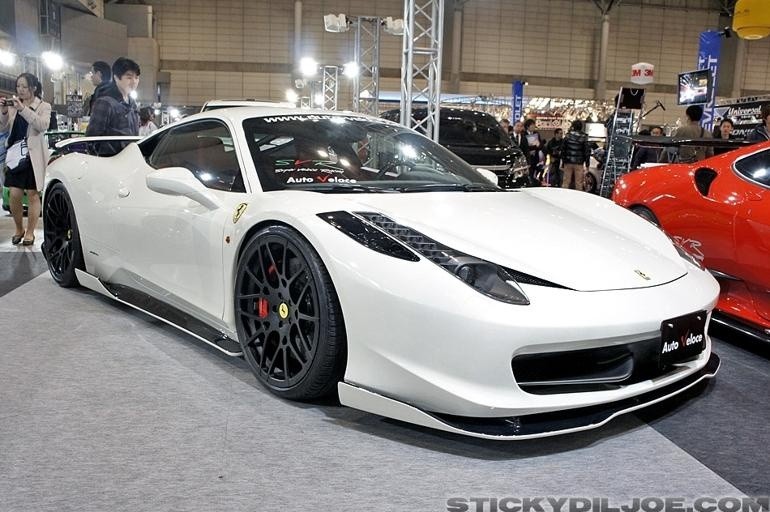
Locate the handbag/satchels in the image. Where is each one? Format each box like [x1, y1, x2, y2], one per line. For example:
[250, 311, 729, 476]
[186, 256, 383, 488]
[2, 140, 31, 178]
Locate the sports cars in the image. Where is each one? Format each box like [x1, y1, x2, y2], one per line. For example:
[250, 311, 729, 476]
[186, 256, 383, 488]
[611, 134, 770, 349]
[39, 104, 719, 445]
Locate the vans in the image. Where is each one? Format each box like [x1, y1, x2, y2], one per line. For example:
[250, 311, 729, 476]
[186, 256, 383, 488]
[376, 106, 533, 190]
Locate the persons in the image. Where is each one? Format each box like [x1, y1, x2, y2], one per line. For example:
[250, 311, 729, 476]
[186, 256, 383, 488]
[0, 73, 52, 244]
[744, 100, 770, 146]
[630, 106, 736, 173]
[86, 58, 159, 161]
[499, 112, 602, 191]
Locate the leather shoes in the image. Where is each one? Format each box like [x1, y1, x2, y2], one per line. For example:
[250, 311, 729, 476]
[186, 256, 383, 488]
[22, 235, 33, 245]
[12, 230, 24, 244]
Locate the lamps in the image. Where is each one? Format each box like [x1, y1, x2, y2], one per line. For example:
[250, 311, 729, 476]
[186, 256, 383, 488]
[323, 13, 351, 33]
[295, 77, 305, 89]
[383, 16, 405, 36]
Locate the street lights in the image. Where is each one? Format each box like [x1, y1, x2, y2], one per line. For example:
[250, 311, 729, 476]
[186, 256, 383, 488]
[287, 56, 359, 115]
[323, 10, 405, 117]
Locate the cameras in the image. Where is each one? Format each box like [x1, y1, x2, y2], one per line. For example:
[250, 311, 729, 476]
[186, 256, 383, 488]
[0, 99, 18, 106]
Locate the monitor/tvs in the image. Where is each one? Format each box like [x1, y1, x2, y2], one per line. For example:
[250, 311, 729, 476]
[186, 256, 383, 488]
[677, 68, 713, 105]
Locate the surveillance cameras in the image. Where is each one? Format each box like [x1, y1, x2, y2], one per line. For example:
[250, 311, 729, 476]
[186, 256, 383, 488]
[724, 26, 732, 39]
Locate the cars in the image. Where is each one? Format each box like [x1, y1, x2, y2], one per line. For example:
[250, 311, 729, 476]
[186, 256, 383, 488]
[200, 97, 298, 115]
[581, 151, 605, 194]
[1, 127, 87, 217]
